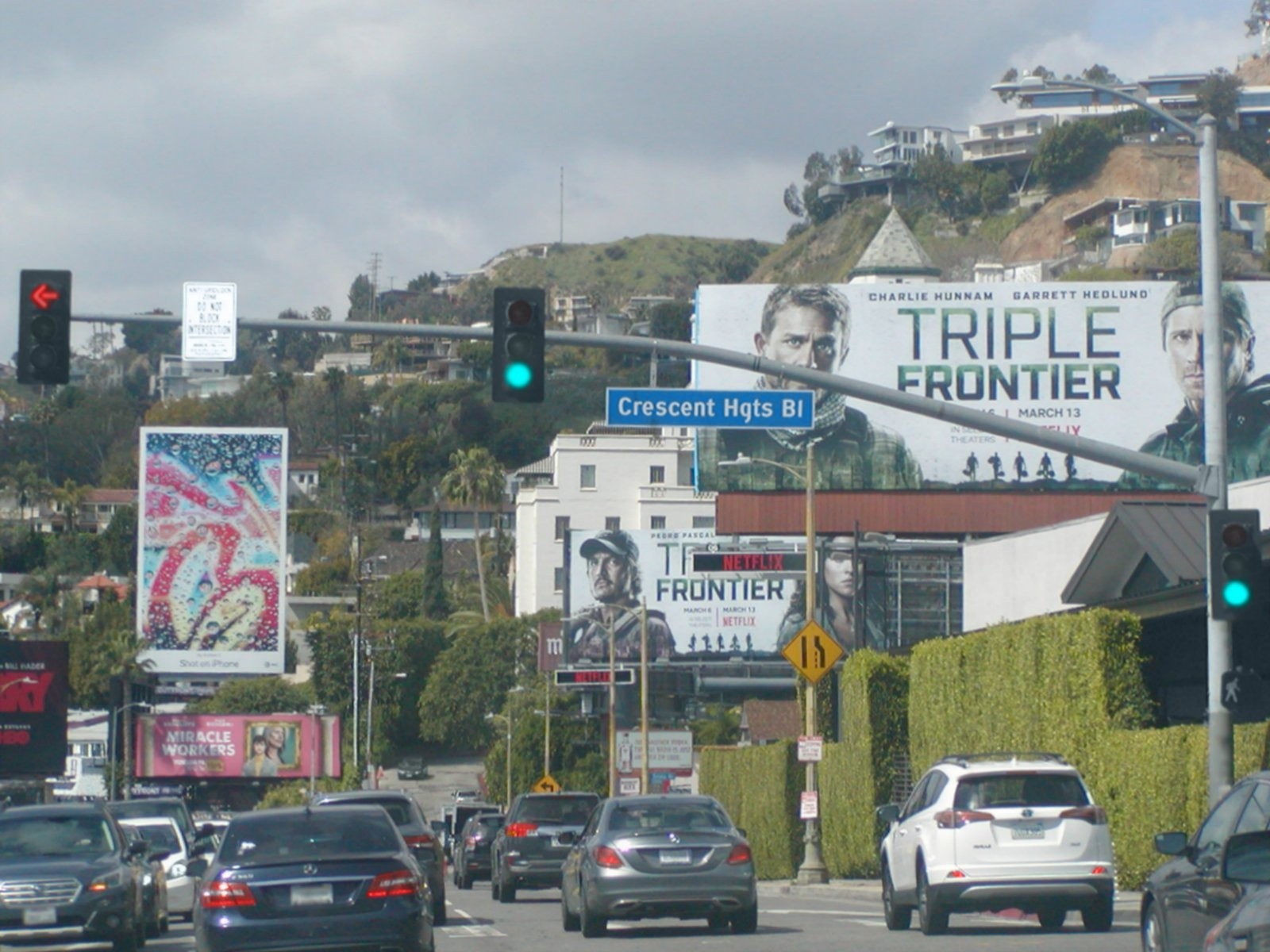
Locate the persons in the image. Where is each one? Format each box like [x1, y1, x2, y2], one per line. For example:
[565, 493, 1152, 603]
[241, 725, 287, 776]
[779, 531, 887, 651]
[1121, 279, 1270, 490]
[570, 529, 678, 663]
[697, 286, 923, 492]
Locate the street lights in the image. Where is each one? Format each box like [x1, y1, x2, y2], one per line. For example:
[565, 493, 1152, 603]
[715, 439, 828, 885]
[558, 588, 651, 798]
[107, 700, 153, 798]
[483, 712, 513, 808]
[510, 670, 552, 777]
[989, 70, 1242, 801]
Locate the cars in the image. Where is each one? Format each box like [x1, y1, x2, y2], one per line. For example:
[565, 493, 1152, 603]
[1135, 769, 1270, 951]
[554, 790, 760, 938]
[71, 823, 171, 940]
[115, 816, 196, 923]
[185, 802, 433, 952]
[398, 758, 428, 779]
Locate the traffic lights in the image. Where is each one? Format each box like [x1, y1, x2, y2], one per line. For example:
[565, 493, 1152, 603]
[489, 284, 550, 404]
[15, 267, 72, 385]
[1204, 506, 1263, 623]
[1217, 665, 1252, 711]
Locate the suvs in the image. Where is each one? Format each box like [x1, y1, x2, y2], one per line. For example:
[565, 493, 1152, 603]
[449, 786, 481, 807]
[486, 788, 602, 905]
[448, 813, 505, 890]
[1, 798, 150, 951]
[316, 791, 447, 924]
[106, 797, 197, 855]
[875, 750, 1115, 936]
